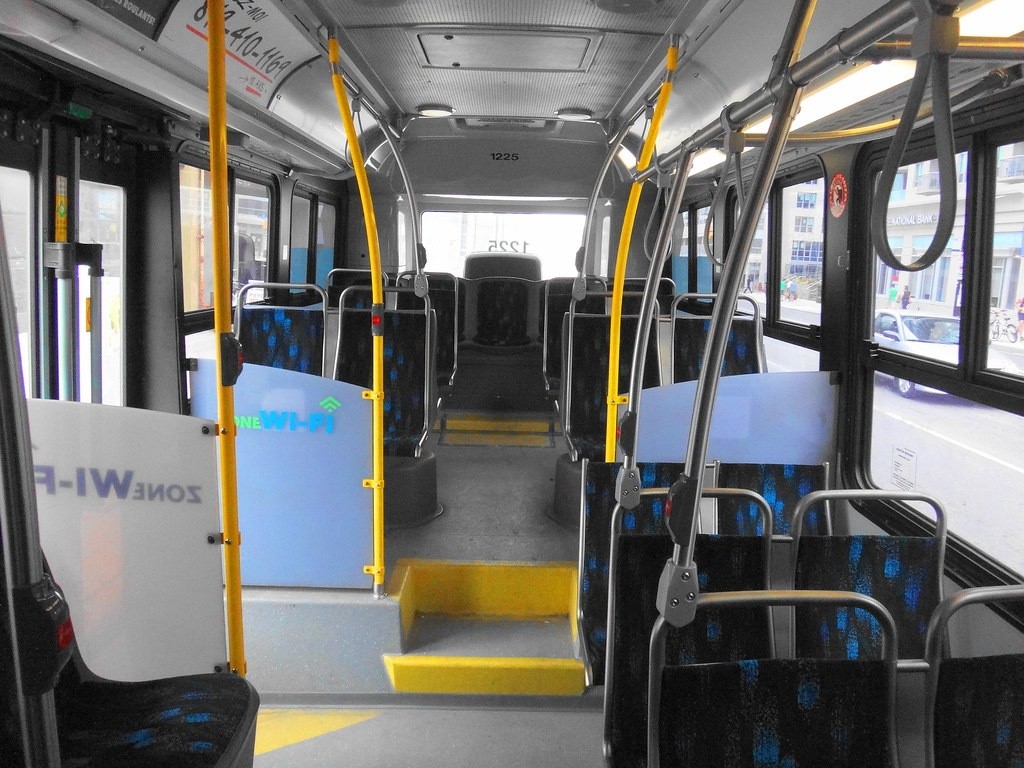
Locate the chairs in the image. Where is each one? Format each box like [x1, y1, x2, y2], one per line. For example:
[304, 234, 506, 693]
[0, 270, 1024, 768]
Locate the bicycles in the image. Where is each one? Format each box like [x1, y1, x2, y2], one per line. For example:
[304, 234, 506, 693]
[990, 310, 1017, 343]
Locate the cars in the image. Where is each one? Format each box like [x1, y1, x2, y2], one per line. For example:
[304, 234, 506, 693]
[874, 309, 960, 398]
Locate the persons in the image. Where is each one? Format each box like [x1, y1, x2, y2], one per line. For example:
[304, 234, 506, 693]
[902, 286, 910, 309]
[1018, 298, 1024, 341]
[887, 283, 896, 307]
[743, 276, 753, 293]
[780, 278, 797, 301]
